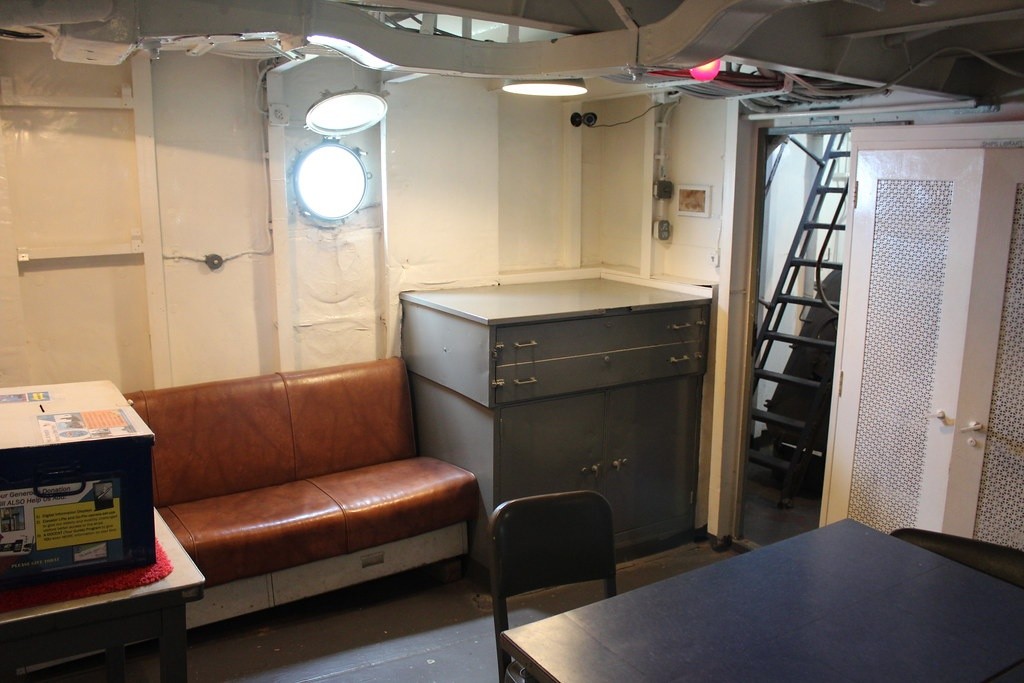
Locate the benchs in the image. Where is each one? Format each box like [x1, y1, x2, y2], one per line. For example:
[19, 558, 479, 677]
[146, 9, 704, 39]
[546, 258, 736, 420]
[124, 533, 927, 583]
[122, 356, 478, 628]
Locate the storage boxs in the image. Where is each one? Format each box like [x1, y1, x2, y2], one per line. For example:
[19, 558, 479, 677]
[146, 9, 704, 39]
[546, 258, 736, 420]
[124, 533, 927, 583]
[0, 380, 155, 588]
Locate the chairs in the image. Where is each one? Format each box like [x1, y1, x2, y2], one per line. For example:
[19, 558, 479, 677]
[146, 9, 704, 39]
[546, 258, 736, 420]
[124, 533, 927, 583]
[488, 489, 617, 683]
[889, 527, 1024, 589]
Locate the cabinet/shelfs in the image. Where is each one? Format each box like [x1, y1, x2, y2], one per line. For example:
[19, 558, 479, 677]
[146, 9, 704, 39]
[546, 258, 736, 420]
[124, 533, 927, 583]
[401, 300, 711, 593]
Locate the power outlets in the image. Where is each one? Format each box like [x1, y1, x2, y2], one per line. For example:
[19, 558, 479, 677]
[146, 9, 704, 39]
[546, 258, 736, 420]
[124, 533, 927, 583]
[653, 220, 670, 241]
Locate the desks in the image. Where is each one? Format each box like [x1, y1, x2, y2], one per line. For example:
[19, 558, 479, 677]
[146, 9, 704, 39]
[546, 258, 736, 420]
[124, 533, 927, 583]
[0, 505, 205, 683]
[498, 517, 1024, 683]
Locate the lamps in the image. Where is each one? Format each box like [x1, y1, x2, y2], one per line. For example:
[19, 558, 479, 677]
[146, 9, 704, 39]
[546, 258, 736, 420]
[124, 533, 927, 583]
[687, 58, 721, 80]
[502, 77, 588, 96]
